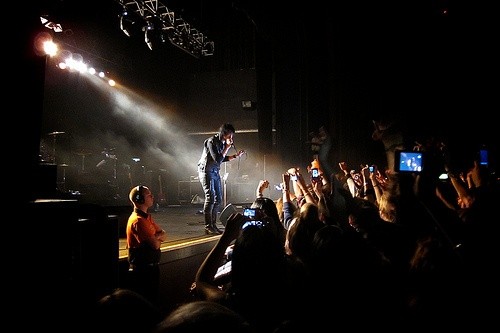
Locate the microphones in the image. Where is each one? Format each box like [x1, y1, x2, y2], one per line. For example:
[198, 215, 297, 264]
[231, 143, 236, 148]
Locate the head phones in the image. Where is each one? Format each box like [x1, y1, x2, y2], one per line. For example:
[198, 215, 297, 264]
[135, 185, 145, 204]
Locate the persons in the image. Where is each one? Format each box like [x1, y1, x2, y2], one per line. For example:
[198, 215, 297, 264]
[198, 123, 245, 235]
[89, 140, 500, 333]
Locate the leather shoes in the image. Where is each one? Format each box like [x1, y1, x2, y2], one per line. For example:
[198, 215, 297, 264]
[205, 225, 216, 235]
[212, 226, 224, 235]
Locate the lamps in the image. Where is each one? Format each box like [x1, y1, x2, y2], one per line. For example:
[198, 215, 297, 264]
[142, 20, 166, 56]
[118, 11, 135, 38]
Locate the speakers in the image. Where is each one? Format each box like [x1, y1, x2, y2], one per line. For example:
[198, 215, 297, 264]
[31, 199, 119, 333]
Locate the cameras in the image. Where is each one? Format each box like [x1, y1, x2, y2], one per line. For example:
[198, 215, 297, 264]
[394, 149, 425, 172]
[312, 168, 318, 180]
[243, 208, 260, 217]
[369, 167, 375, 172]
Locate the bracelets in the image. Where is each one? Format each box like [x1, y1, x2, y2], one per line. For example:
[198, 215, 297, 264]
[233, 155, 236, 158]
